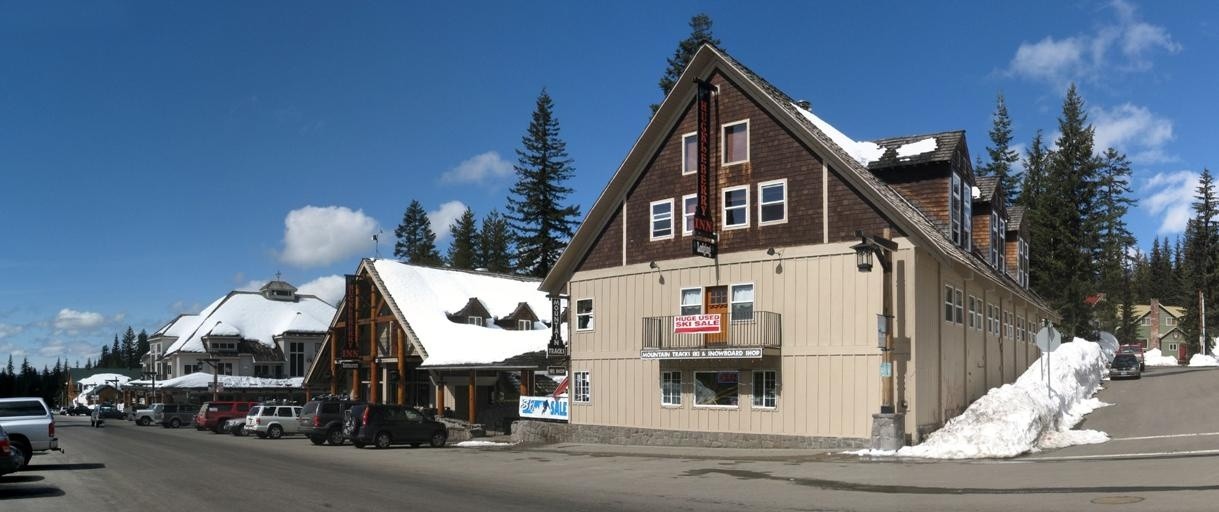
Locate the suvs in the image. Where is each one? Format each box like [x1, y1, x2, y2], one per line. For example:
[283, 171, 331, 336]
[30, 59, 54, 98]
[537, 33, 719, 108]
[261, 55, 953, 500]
[1114, 342, 1148, 370]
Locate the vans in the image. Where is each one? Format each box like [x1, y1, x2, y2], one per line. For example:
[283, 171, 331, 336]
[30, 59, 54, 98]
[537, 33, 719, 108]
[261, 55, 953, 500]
[0, 398, 65, 471]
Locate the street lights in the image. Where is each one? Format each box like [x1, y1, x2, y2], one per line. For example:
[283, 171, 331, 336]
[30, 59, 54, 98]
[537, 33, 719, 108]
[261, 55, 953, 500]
[196, 356, 220, 404]
[850, 222, 901, 415]
[104, 376, 119, 407]
[84, 381, 97, 409]
[139, 370, 158, 404]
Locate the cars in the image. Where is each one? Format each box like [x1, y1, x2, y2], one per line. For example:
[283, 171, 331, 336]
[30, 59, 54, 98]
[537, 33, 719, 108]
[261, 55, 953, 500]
[0, 424, 25, 473]
[1109, 354, 1141, 381]
